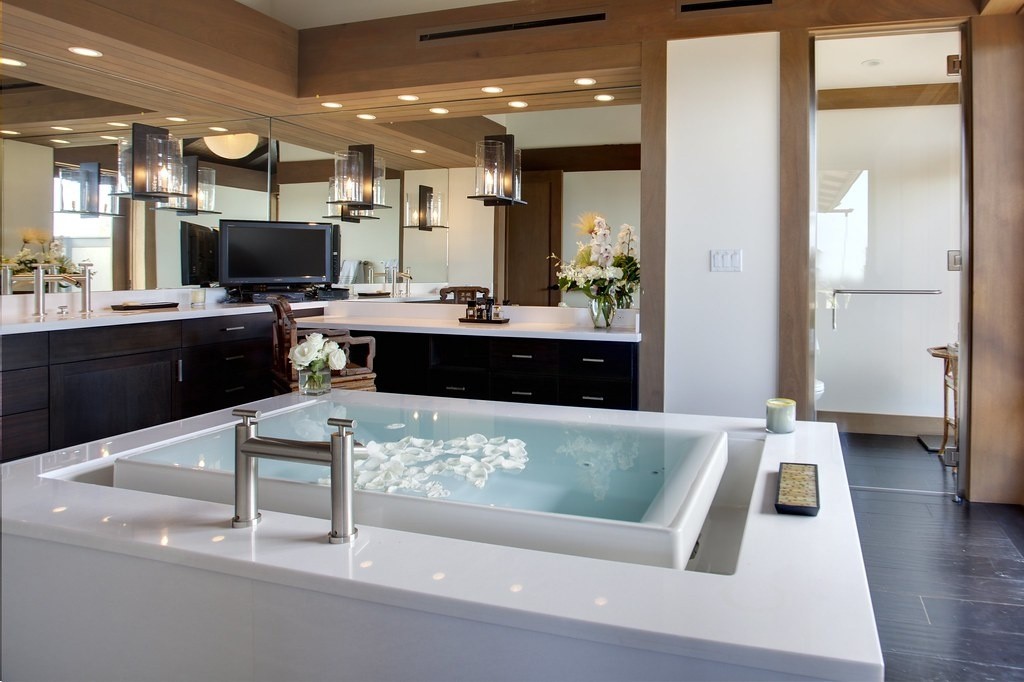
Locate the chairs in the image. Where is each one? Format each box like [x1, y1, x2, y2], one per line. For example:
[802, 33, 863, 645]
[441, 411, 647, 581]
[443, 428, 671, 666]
[266, 296, 376, 394]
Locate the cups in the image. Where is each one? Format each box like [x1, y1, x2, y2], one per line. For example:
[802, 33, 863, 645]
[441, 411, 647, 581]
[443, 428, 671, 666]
[766, 397, 796, 433]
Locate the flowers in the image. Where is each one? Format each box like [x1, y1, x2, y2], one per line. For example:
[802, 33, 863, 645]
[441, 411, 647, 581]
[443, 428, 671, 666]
[289, 332, 346, 387]
[0, 227, 96, 290]
[545, 215, 632, 320]
[606, 225, 640, 307]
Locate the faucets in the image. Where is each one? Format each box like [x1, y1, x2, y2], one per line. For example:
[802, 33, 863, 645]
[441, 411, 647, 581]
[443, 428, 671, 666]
[368, 266, 390, 283]
[0, 262, 61, 295]
[392, 267, 412, 297]
[30, 263, 94, 315]
[231, 406, 370, 545]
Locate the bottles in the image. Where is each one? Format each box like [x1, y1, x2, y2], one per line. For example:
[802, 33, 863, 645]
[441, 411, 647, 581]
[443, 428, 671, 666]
[465, 299, 501, 320]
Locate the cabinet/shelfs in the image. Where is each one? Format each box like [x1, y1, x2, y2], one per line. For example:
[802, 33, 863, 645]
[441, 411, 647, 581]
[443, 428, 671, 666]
[0, 308, 638, 464]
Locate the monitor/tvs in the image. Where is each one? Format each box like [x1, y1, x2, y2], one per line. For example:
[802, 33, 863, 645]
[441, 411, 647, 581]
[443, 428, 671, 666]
[332, 225, 341, 284]
[180, 221, 220, 289]
[217, 219, 333, 292]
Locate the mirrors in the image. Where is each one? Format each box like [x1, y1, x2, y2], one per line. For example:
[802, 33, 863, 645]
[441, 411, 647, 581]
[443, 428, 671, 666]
[0, 43, 272, 294]
[266, 83, 642, 308]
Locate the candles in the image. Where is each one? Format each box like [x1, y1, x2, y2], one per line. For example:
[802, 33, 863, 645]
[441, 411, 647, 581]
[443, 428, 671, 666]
[766, 398, 796, 434]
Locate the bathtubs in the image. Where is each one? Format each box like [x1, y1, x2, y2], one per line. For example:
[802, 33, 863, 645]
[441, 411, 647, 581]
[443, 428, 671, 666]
[116, 399, 729, 570]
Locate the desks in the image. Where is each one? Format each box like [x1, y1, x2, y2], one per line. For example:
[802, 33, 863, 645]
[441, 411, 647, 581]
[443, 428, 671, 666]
[926, 346, 960, 472]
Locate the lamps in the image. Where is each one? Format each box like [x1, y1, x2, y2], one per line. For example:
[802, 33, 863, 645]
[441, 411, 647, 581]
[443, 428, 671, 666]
[326, 142, 374, 208]
[321, 156, 391, 224]
[419, 185, 449, 232]
[51, 133, 222, 218]
[131, 123, 191, 198]
[483, 135, 528, 207]
[204, 132, 260, 159]
[467, 135, 516, 201]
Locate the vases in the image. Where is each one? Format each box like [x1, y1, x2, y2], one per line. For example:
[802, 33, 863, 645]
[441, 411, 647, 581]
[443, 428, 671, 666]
[613, 286, 634, 311]
[298, 363, 331, 396]
[586, 282, 614, 329]
